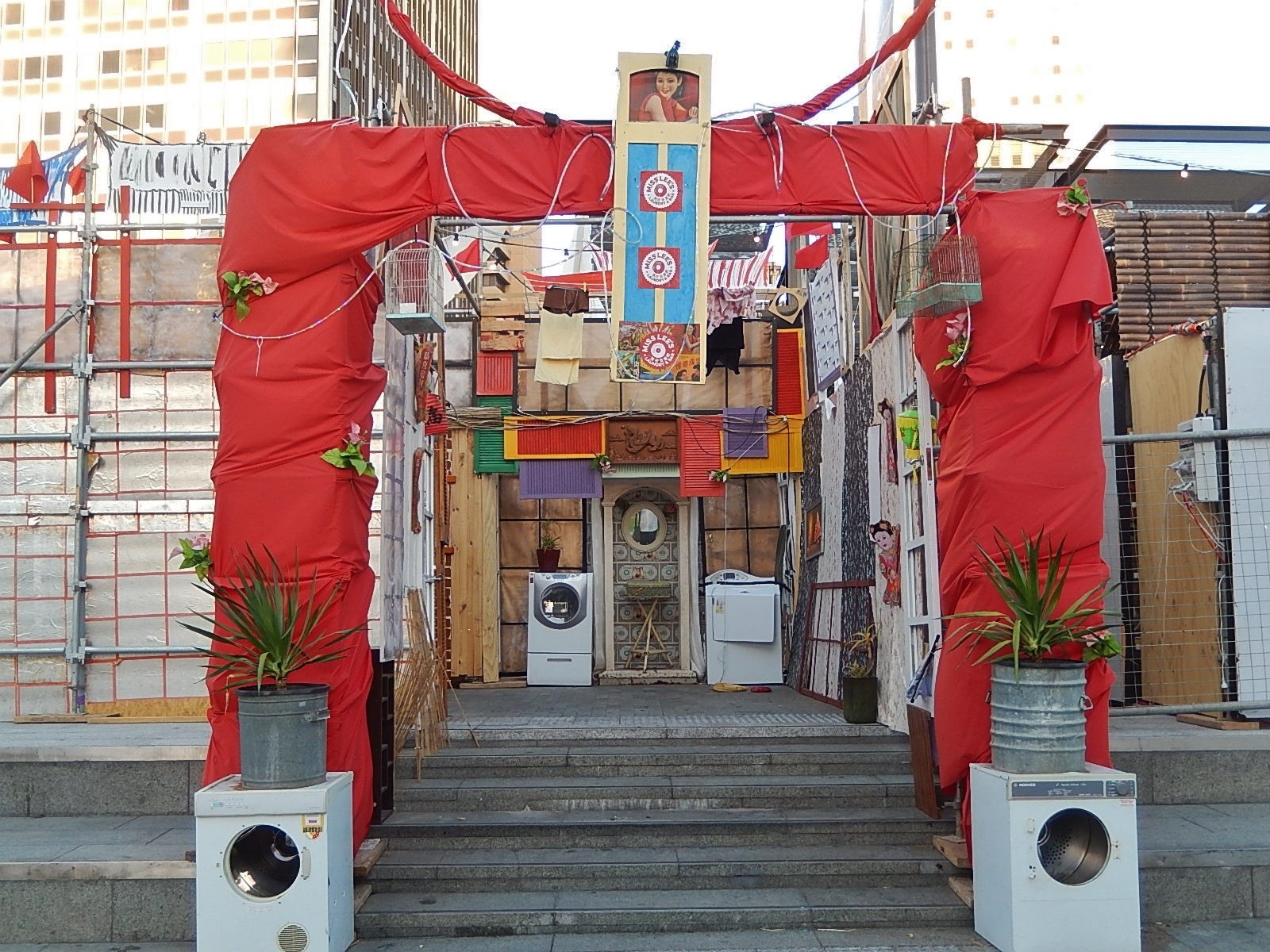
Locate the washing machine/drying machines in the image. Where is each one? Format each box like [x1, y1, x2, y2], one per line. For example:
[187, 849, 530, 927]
[705, 570, 786, 684]
[967, 758, 1142, 952]
[528, 573, 594, 687]
[193, 766, 355, 952]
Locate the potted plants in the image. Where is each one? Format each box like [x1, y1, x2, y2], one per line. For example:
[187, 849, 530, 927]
[530, 518, 566, 572]
[928, 523, 1130, 775]
[170, 541, 382, 791]
[841, 622, 882, 725]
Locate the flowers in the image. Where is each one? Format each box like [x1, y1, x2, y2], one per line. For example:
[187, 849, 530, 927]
[930, 307, 974, 376]
[1066, 177, 1090, 206]
[320, 420, 376, 478]
[707, 467, 731, 483]
[166, 530, 216, 581]
[211, 271, 280, 326]
[1081, 625, 1122, 665]
[592, 450, 617, 476]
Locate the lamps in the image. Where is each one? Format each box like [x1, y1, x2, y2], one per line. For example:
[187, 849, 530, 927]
[1241, 200, 1270, 213]
[544, 112, 561, 137]
[754, 113, 776, 136]
[664, 40, 681, 67]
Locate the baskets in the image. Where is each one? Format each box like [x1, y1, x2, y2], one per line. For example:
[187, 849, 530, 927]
[894, 233, 984, 318]
